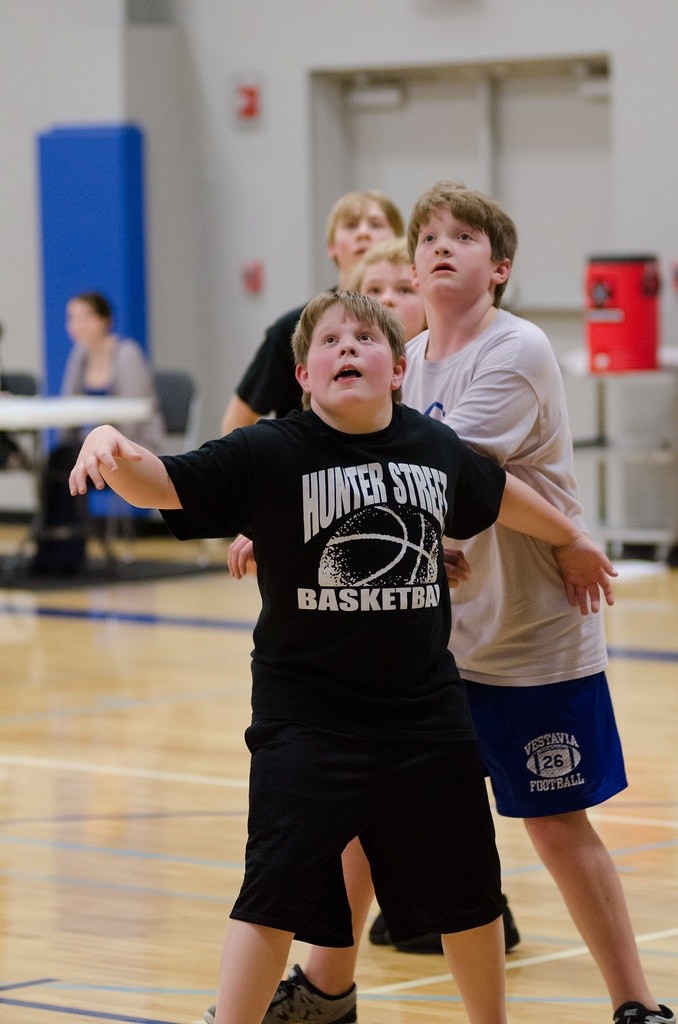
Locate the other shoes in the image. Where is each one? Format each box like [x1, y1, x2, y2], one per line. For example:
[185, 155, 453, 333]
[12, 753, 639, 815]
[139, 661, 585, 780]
[397, 894, 520, 951]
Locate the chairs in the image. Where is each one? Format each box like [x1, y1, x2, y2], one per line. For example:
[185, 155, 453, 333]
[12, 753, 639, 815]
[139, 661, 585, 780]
[16, 372, 194, 575]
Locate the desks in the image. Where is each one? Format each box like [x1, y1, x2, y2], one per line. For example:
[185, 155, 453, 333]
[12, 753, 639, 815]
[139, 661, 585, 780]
[0, 393, 153, 582]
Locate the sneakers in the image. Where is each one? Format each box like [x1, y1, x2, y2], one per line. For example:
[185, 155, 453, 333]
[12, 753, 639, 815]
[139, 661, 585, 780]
[612, 1001, 675, 1024]
[205, 963, 358, 1024]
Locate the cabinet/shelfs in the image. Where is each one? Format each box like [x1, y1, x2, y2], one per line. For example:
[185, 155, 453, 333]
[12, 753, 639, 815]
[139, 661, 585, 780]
[568, 350, 678, 560]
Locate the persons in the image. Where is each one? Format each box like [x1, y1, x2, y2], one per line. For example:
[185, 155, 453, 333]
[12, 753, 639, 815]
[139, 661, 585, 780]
[219, 188, 407, 437]
[206, 179, 678, 1024]
[20, 292, 167, 574]
[67, 285, 619, 1024]
[349, 235, 521, 960]
